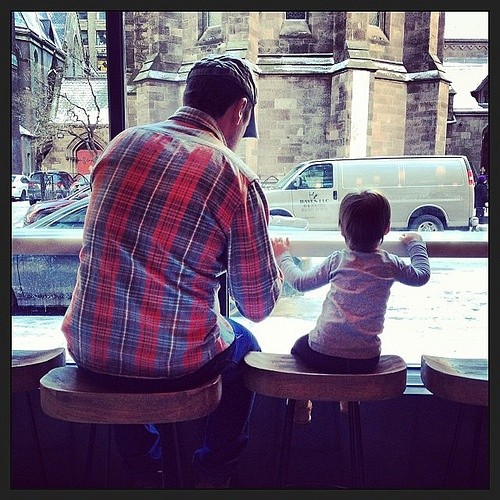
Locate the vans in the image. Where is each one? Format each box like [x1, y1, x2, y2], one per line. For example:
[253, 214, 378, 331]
[261, 154, 479, 231]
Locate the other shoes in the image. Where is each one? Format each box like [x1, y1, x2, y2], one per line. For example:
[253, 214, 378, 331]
[193, 476, 231, 491]
[293, 400, 312, 423]
[339, 399, 363, 413]
[131, 470, 164, 490]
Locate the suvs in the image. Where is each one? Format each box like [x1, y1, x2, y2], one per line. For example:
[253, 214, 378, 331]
[29, 170, 74, 206]
[24, 184, 91, 226]
[12, 174, 29, 201]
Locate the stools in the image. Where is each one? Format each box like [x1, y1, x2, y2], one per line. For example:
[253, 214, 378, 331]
[243, 350, 406, 489]
[40, 366, 222, 489]
[11, 347, 64, 488]
[421, 355, 488, 489]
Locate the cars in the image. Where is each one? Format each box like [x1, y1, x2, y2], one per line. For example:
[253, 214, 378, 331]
[69, 174, 92, 194]
[26, 197, 90, 230]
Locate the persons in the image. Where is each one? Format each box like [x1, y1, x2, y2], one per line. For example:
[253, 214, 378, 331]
[270, 188, 431, 425]
[61, 52, 285, 463]
[471, 175, 488, 232]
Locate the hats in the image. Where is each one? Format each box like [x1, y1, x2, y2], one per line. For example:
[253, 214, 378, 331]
[186, 55, 259, 140]
[479, 175, 486, 182]
